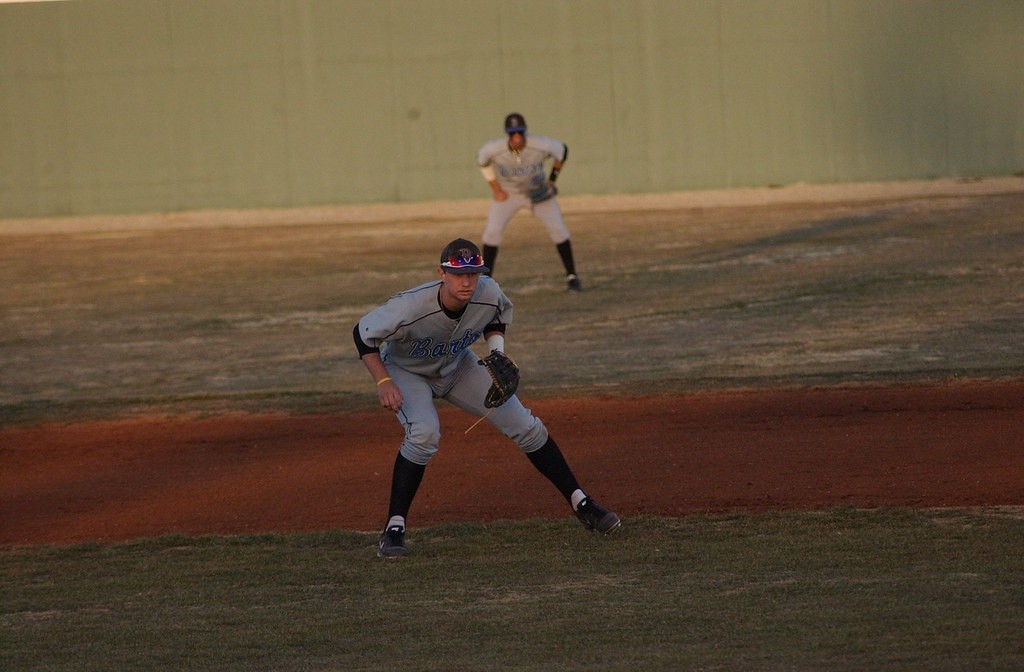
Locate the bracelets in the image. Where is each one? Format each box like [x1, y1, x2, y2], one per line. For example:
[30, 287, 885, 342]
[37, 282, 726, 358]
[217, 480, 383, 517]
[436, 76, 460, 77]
[376, 377, 392, 385]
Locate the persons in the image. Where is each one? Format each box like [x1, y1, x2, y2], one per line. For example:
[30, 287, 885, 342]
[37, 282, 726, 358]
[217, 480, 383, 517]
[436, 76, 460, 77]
[353, 237, 620, 557]
[474, 113, 581, 293]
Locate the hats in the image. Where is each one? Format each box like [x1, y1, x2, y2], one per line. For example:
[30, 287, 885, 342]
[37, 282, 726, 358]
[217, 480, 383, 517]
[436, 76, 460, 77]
[503, 111, 526, 134]
[439, 237, 490, 275]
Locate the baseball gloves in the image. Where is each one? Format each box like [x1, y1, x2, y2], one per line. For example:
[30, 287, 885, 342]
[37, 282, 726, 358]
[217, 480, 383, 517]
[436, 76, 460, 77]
[477, 348, 520, 408]
[529, 184, 557, 203]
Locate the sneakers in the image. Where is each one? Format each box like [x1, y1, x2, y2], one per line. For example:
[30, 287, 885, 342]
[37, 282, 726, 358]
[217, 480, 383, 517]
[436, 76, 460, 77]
[573, 494, 621, 537]
[376, 524, 410, 560]
[565, 273, 579, 291]
